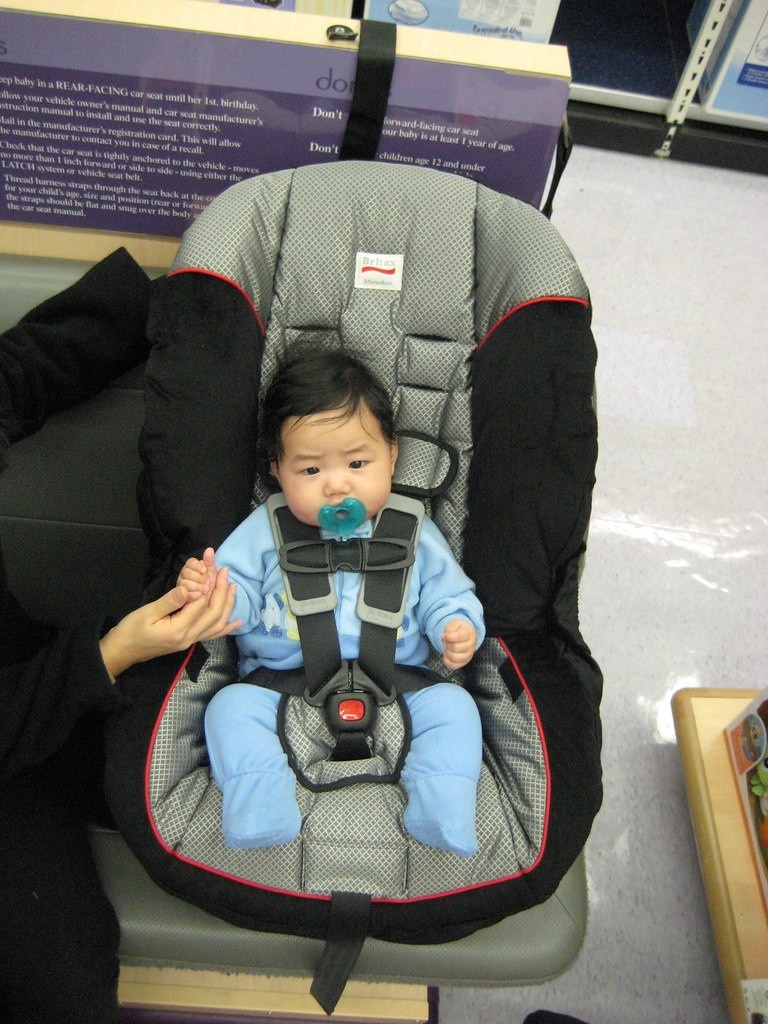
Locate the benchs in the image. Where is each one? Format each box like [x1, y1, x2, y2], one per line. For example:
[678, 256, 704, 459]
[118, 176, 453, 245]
[0, 253, 588, 994]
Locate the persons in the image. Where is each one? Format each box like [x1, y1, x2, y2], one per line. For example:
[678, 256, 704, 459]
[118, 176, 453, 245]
[0, 246, 245, 1024]
[175, 350, 486, 857]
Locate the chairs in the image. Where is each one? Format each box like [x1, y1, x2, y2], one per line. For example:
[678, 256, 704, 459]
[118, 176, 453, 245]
[79, 156, 604, 947]
